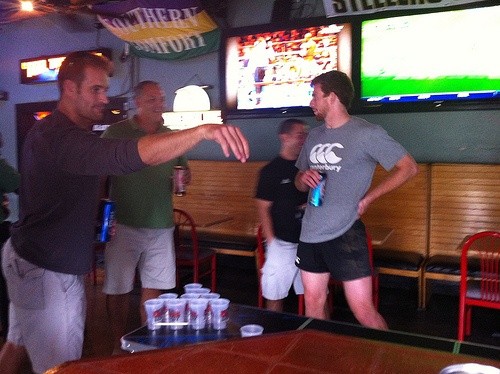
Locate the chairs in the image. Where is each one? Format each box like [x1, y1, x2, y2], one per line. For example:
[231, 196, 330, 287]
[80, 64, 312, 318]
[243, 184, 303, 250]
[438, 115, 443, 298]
[172, 208, 217, 292]
[328, 227, 378, 312]
[256, 224, 303, 317]
[457, 231, 500, 342]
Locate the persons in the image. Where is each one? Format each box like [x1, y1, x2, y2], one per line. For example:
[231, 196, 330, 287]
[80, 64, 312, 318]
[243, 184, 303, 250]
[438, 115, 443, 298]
[0, 51, 250, 374]
[293, 72, 420, 330]
[253, 119, 329, 314]
[237, 25, 337, 105]
[100, 81, 192, 354]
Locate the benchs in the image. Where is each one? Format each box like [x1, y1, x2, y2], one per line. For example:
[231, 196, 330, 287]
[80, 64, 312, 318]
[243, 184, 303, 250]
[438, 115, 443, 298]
[172, 160, 500, 312]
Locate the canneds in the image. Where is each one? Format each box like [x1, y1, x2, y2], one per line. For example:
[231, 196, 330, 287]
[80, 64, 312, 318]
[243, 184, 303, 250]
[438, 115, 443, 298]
[309, 170, 327, 207]
[174, 165, 187, 197]
[96, 199, 115, 243]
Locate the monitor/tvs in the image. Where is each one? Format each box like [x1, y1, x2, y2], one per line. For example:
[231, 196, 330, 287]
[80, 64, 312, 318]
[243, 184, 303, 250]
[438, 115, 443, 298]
[217, 0, 500, 120]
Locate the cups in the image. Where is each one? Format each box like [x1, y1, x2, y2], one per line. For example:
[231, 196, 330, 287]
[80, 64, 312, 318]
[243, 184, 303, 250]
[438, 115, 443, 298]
[190, 299, 208, 330]
[159, 295, 177, 324]
[182, 293, 200, 323]
[188, 289, 211, 294]
[200, 294, 220, 325]
[168, 299, 185, 330]
[185, 284, 202, 293]
[144, 299, 163, 330]
[240, 325, 264, 337]
[210, 298, 230, 330]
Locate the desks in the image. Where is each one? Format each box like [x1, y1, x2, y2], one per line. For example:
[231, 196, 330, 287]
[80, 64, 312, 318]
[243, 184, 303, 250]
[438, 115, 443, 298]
[45, 303, 500, 374]
